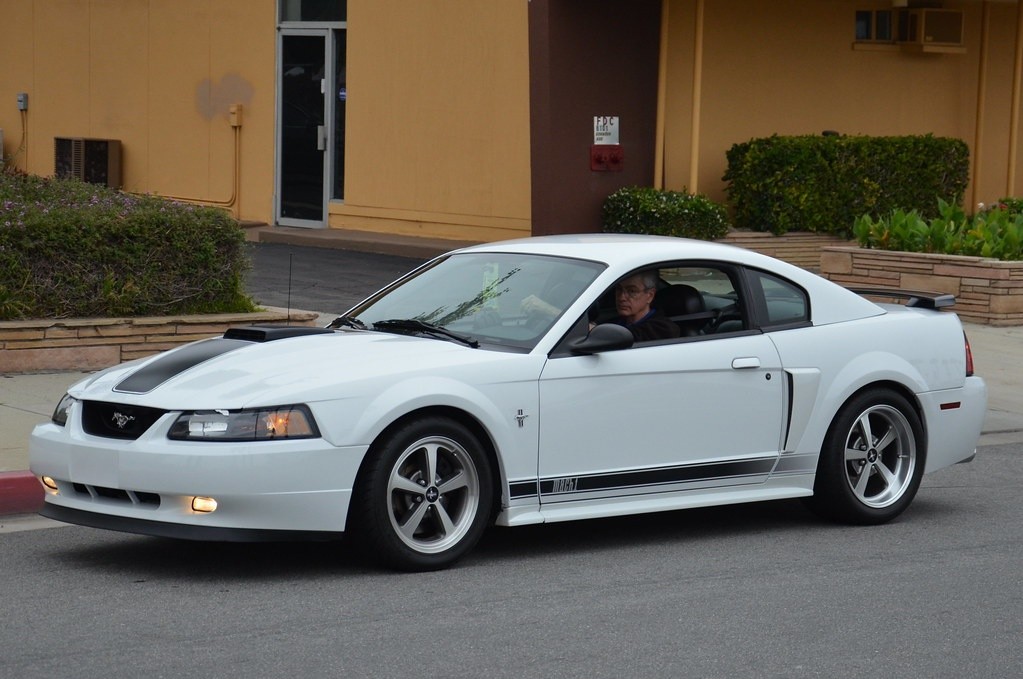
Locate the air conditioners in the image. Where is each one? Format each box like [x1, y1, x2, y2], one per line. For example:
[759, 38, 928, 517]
[900, 9, 966, 46]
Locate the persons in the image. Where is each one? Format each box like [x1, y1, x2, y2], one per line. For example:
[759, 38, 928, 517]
[520, 273, 679, 341]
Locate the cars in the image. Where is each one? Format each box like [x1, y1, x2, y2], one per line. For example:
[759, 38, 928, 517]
[26, 232, 992, 572]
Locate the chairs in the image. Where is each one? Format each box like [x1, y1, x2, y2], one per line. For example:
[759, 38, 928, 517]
[654, 284, 706, 337]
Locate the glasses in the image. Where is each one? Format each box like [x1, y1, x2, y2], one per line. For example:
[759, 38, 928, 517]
[616, 286, 648, 296]
